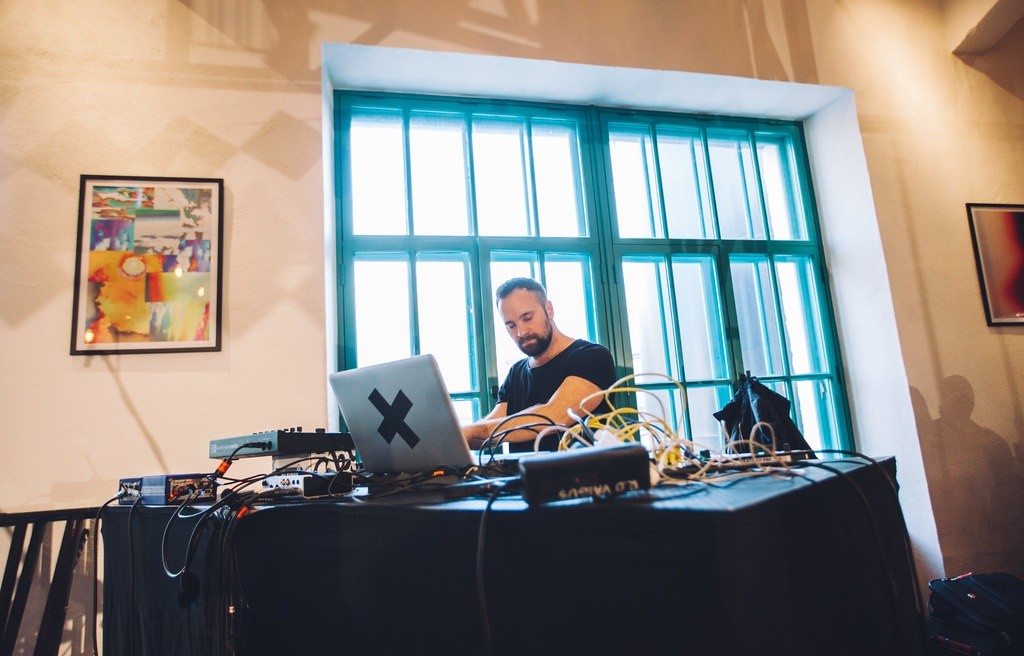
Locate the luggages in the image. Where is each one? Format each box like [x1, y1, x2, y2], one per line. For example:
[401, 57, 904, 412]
[924, 613, 1024, 656]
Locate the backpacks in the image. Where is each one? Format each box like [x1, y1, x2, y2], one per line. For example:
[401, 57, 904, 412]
[928, 572, 1024, 634]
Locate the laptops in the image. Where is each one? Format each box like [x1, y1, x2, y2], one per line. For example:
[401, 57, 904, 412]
[329, 353, 476, 476]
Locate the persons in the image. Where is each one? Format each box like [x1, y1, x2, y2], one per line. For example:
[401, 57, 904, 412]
[460, 277, 617, 453]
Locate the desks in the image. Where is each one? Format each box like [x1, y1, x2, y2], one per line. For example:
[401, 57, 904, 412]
[101, 457, 919, 656]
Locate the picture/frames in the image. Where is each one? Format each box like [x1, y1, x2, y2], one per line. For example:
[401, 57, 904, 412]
[69, 174, 224, 356]
[966, 203, 1024, 327]
